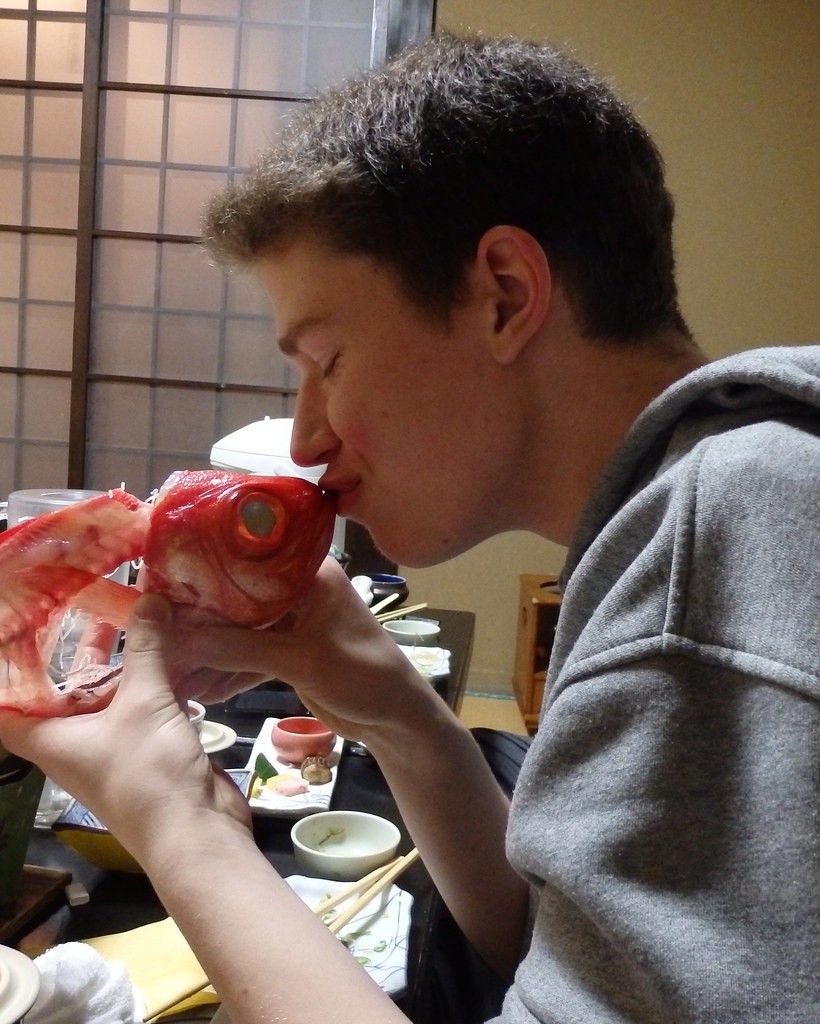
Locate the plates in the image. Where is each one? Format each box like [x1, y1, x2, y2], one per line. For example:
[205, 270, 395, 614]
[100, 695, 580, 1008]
[243, 717, 344, 817]
[200, 720, 236, 754]
[283, 874, 416, 998]
[397, 644, 451, 682]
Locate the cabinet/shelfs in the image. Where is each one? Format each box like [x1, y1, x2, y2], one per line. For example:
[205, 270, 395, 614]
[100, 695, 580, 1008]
[510, 575, 563, 732]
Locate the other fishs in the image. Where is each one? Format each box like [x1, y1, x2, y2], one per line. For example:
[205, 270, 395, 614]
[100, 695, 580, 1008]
[0, 469, 337, 718]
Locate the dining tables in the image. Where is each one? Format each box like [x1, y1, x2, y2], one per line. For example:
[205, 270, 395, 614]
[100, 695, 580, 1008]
[0, 610, 480, 1024]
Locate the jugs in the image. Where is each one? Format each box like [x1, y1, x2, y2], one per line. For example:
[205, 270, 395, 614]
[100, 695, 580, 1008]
[6, 490, 131, 680]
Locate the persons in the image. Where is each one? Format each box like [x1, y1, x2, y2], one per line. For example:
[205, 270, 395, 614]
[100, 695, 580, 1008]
[0, 32, 820, 1021]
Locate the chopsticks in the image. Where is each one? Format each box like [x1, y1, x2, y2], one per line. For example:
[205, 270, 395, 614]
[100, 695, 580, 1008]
[376, 602, 428, 622]
[141, 847, 419, 1024]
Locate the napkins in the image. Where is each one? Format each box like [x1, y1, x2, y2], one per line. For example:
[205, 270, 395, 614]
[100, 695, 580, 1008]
[285, 874, 414, 997]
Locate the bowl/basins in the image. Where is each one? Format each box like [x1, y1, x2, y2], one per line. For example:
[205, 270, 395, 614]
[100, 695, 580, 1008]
[187, 700, 206, 742]
[271, 717, 337, 763]
[51, 767, 255, 873]
[351, 573, 409, 607]
[291, 809, 401, 881]
[383, 620, 442, 645]
